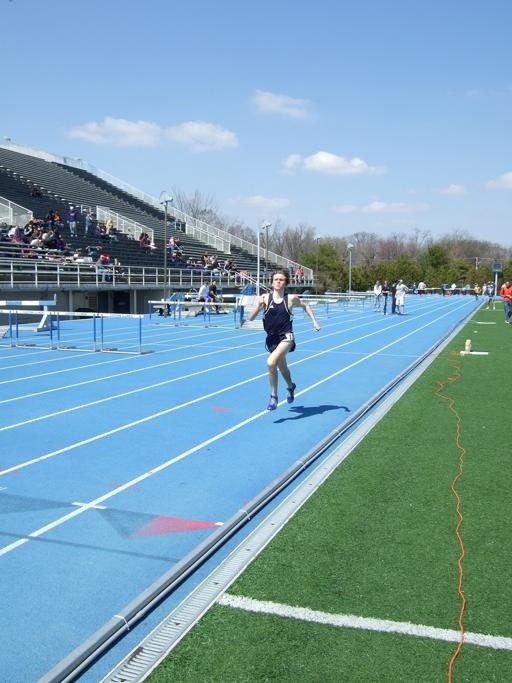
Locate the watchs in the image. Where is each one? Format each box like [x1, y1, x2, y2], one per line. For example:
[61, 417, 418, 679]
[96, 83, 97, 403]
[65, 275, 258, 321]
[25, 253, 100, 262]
[372, 280, 382, 311]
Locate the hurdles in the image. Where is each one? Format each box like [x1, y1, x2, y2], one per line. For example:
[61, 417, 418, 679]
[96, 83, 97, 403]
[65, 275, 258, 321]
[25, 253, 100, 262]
[0, 292, 159, 357]
[406, 286, 478, 297]
[147, 281, 397, 329]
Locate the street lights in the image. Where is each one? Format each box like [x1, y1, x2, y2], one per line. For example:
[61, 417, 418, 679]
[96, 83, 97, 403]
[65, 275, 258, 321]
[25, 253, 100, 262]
[314, 232, 320, 298]
[261, 218, 271, 293]
[346, 243, 353, 292]
[159, 189, 174, 319]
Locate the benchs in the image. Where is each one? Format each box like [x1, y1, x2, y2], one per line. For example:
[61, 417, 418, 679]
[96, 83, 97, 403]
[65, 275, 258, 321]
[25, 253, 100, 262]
[2, 146, 301, 277]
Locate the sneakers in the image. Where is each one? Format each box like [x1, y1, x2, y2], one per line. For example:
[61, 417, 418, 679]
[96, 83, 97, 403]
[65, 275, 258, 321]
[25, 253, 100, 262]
[287, 383, 296, 404]
[267, 395, 279, 410]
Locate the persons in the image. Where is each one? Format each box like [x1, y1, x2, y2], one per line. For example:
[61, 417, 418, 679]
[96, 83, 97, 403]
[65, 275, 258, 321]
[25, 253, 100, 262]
[208, 276, 226, 314]
[140, 231, 150, 254]
[499, 280, 512, 327]
[2, 203, 120, 285]
[382, 279, 388, 316]
[412, 277, 485, 301]
[394, 278, 409, 314]
[197, 281, 213, 313]
[389, 278, 397, 313]
[485, 281, 496, 311]
[169, 234, 242, 283]
[247, 268, 321, 412]
[295, 264, 303, 282]
